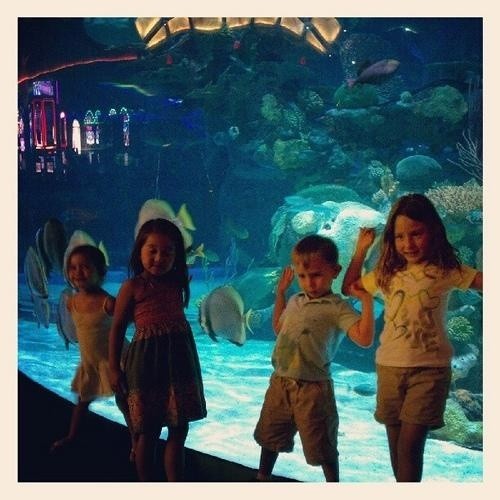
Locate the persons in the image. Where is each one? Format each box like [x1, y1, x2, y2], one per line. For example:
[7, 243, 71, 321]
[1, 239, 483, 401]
[252, 235, 374, 483]
[108, 219, 206, 482]
[342, 195, 483, 482]
[54, 244, 138, 461]
